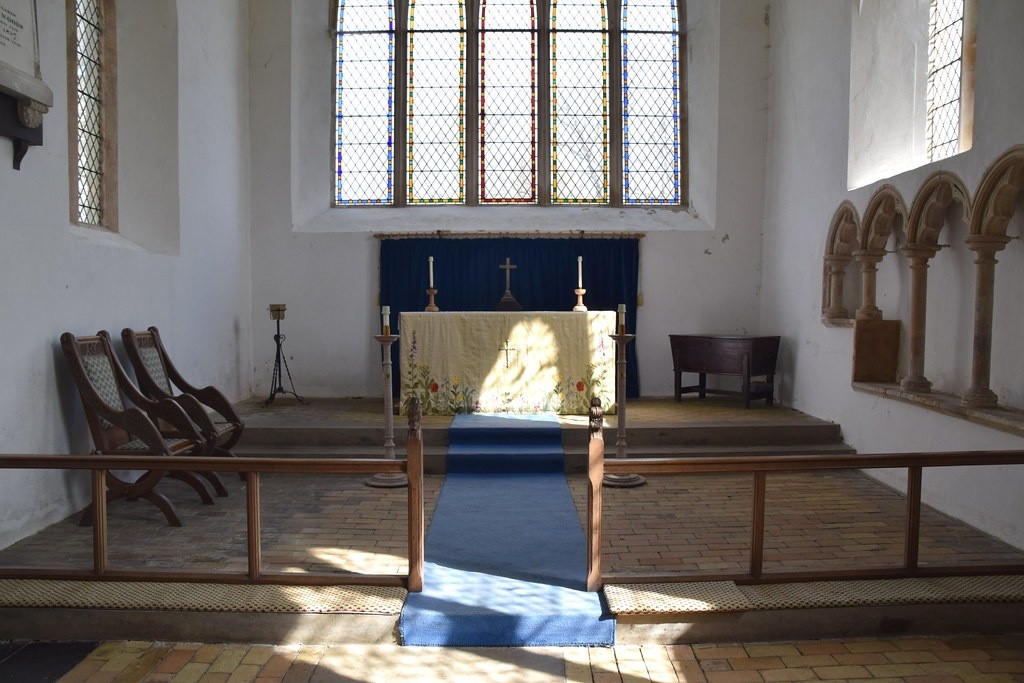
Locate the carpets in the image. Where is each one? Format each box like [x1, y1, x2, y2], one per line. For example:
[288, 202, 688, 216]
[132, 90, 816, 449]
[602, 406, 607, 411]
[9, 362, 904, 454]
[397, 411, 615, 650]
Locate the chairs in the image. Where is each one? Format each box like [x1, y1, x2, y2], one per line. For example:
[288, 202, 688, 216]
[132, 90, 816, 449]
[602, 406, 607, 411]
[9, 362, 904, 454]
[60, 330, 214, 528]
[122, 325, 251, 497]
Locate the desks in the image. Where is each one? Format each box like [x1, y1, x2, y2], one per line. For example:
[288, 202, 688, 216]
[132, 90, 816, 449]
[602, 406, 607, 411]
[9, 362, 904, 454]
[669, 335, 783, 410]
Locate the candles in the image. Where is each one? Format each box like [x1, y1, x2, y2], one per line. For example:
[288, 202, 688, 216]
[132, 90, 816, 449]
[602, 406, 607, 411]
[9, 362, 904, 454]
[381, 305, 391, 331]
[428, 255, 435, 288]
[577, 253, 583, 291]
[505, 256, 511, 291]
[617, 302, 627, 329]
